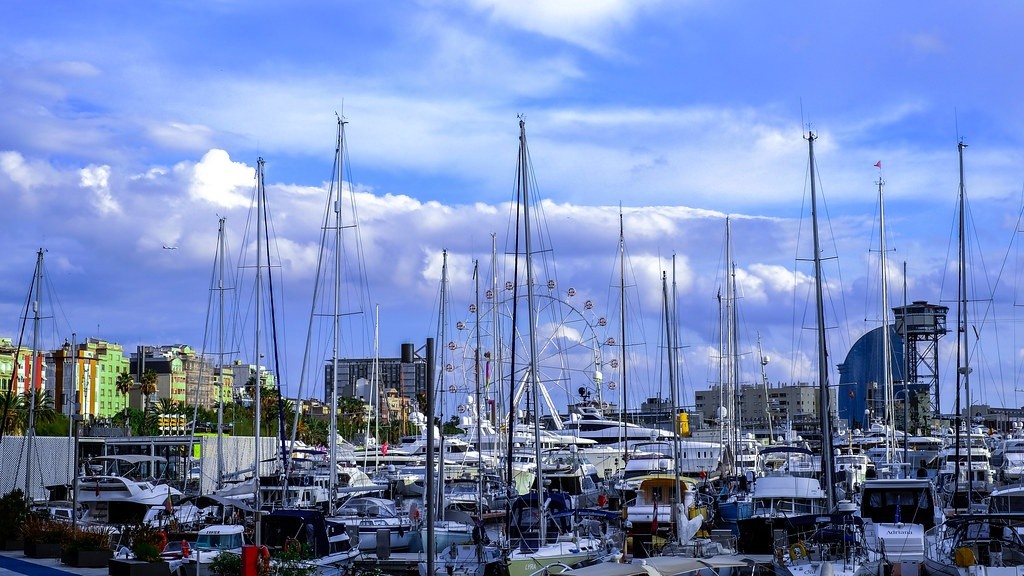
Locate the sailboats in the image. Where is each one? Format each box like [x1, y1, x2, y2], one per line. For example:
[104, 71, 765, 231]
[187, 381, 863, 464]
[0, 91, 1023, 576]
